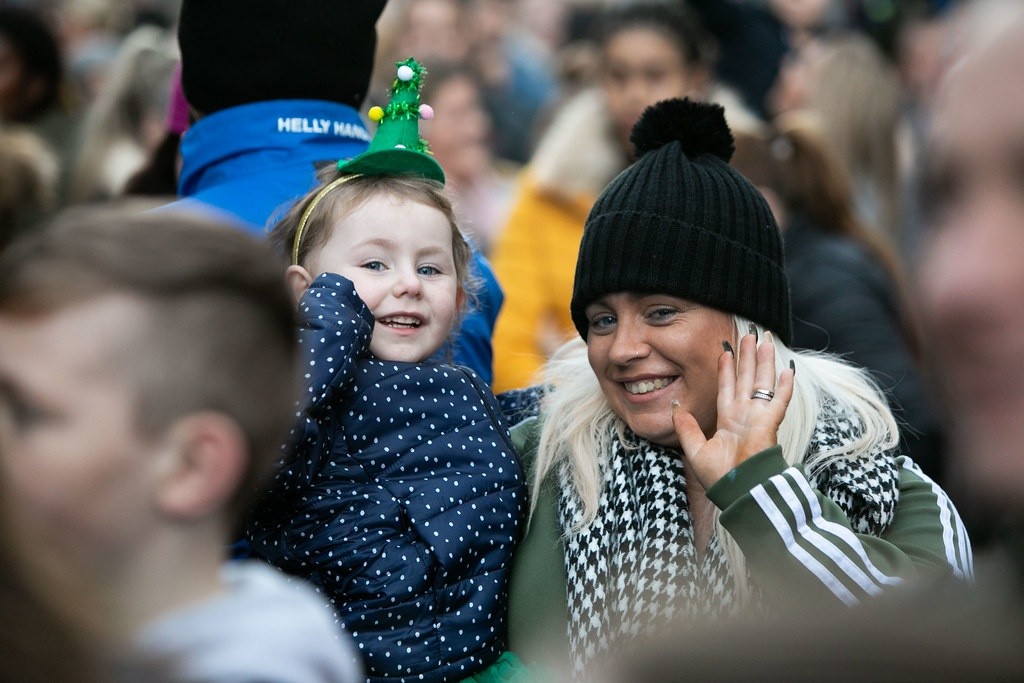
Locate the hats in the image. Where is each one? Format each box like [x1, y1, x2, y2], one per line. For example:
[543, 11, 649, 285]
[572, 99, 796, 351]
[176, 0, 391, 114]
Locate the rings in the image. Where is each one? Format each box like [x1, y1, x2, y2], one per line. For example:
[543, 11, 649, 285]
[751, 388, 774, 401]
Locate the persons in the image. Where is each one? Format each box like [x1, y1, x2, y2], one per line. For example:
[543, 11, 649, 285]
[147, 0, 509, 394]
[503, 95, 973, 683]
[1, 2, 1023, 497]
[1, 195, 362, 683]
[221, 169, 535, 683]
[509, 98, 975, 683]
